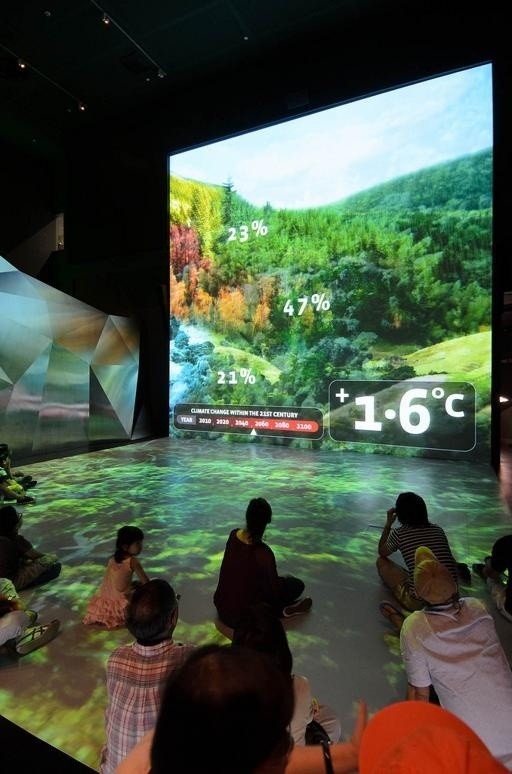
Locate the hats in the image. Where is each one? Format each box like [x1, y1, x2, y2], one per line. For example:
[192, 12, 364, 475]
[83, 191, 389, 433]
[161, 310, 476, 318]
[414, 546, 456, 604]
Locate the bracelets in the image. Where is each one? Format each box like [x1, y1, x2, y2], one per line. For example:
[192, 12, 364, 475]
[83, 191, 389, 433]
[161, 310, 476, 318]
[322, 741, 333, 771]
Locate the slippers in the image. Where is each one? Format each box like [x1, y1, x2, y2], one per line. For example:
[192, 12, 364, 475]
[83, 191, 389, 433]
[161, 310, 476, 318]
[380, 600, 404, 627]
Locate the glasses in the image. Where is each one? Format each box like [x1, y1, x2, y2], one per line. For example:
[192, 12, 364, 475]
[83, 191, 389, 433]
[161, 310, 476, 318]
[17, 513, 22, 521]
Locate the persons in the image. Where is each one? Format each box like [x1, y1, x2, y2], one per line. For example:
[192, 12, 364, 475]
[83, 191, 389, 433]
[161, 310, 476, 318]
[213, 498, 312, 628]
[111, 645, 367, 774]
[82, 525, 149, 630]
[472, 493, 512, 624]
[379, 547, 511, 772]
[0, 576, 61, 655]
[0, 504, 61, 592]
[0, 442, 37, 507]
[221, 604, 342, 745]
[359, 700, 511, 773]
[376, 492, 458, 611]
[99, 580, 193, 774]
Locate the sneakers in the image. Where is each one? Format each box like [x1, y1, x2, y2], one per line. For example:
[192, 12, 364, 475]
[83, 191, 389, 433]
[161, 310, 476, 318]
[16, 618, 60, 656]
[283, 597, 312, 619]
[25, 609, 37, 624]
[29, 481, 37, 487]
[17, 496, 33, 504]
[38, 562, 61, 583]
